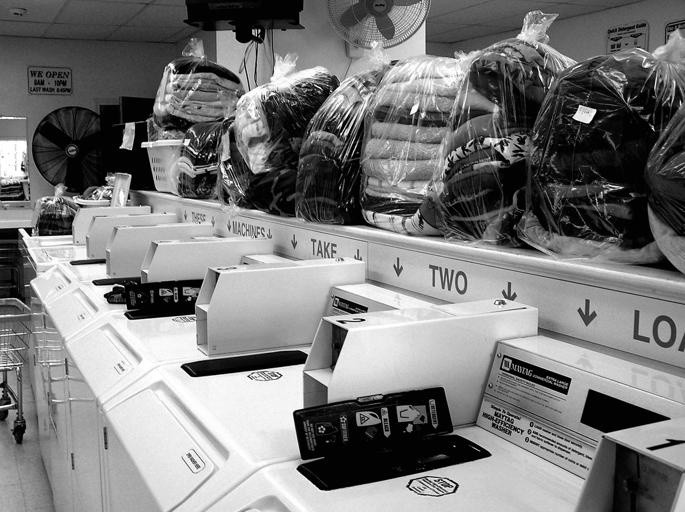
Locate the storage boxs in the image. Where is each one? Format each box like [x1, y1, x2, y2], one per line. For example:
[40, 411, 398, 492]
[110, 118, 153, 191]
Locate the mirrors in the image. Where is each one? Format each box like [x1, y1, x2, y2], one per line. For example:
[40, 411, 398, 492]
[0, 115, 31, 202]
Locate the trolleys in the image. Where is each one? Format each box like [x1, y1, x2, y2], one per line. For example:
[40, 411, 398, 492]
[0, 298, 34, 445]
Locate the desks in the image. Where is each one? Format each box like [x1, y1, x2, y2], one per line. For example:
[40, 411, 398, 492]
[0, 207, 36, 245]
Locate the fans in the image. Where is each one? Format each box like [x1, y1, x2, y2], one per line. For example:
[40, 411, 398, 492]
[324, 0, 431, 58]
[32, 106, 116, 194]
